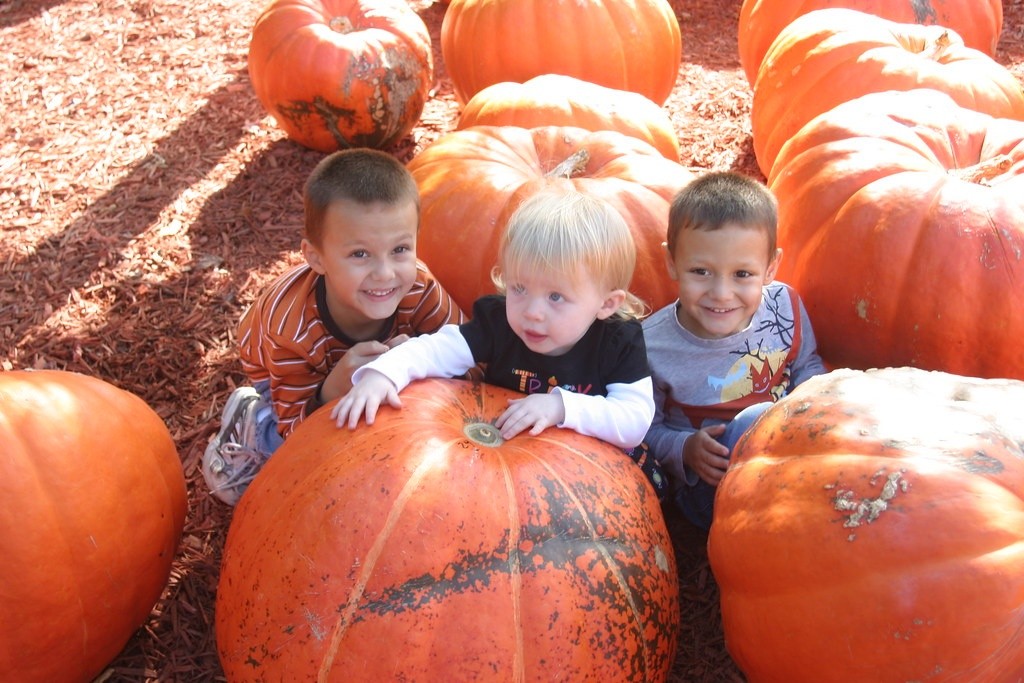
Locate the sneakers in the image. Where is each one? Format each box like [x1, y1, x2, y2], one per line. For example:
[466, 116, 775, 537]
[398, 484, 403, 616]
[203, 386, 269, 507]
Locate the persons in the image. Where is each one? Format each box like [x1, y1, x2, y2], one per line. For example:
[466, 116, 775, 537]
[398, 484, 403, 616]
[640, 171, 829, 544]
[329, 190, 657, 449]
[201, 148, 487, 507]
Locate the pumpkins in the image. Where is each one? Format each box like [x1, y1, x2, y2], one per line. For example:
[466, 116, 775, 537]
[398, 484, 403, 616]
[0, 368, 187, 683]
[217, 0, 1024, 683]
